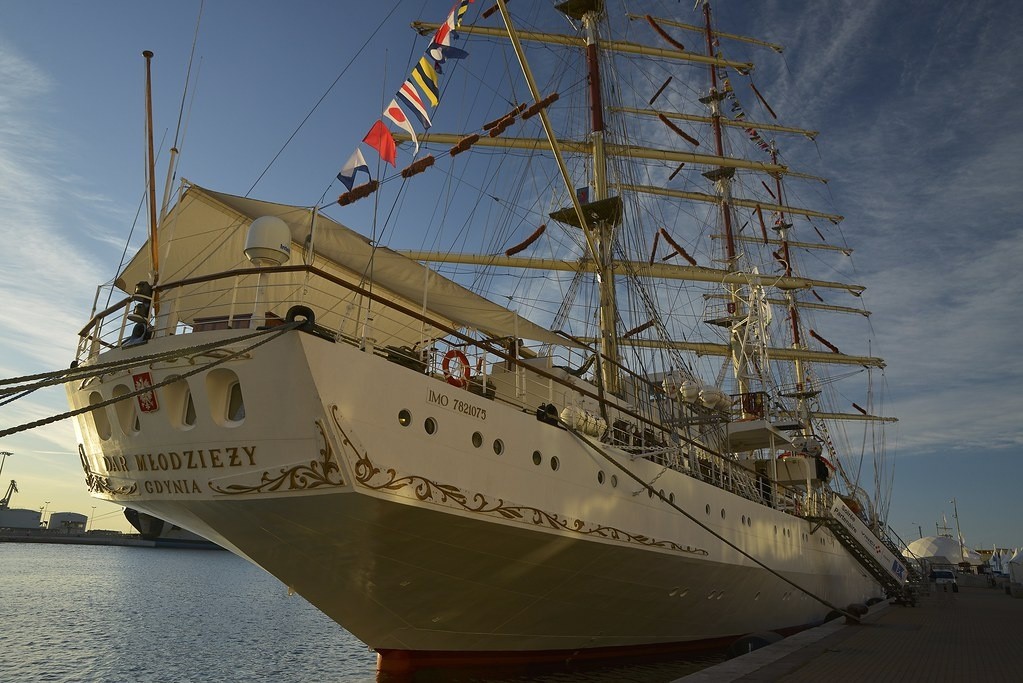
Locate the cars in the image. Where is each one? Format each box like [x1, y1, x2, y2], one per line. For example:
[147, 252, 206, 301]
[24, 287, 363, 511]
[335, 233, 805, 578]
[929, 567, 959, 593]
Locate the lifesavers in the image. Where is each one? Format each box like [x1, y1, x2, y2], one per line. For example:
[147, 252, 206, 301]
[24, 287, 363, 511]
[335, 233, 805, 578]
[441, 349, 471, 388]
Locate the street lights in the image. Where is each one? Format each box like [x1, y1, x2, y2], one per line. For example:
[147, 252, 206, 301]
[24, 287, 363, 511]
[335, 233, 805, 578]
[90, 505, 97, 529]
[42, 501, 51, 526]
[0, 451, 13, 474]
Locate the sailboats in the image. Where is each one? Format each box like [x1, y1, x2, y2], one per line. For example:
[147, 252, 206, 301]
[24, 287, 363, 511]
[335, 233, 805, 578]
[60, 0, 931, 682]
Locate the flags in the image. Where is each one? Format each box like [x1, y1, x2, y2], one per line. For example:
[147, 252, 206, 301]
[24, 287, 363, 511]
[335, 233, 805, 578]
[712, 38, 773, 154]
[337, 1, 472, 192]
